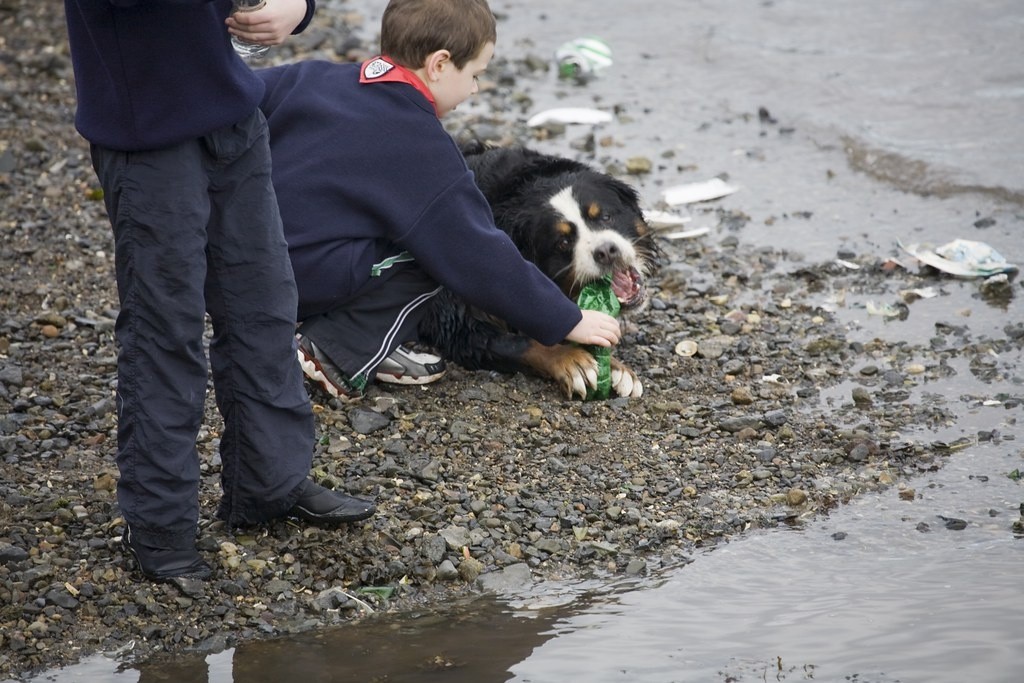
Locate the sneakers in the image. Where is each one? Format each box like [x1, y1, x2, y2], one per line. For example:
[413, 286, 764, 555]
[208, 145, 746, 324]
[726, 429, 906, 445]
[374, 344, 447, 385]
[295, 332, 367, 404]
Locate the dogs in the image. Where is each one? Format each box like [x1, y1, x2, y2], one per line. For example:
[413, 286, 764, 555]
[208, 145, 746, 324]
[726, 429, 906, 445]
[418, 129, 666, 401]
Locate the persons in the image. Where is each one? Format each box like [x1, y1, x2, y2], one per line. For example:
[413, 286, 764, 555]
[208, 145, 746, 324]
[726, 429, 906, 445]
[64, 0, 376, 578]
[256, 0, 621, 405]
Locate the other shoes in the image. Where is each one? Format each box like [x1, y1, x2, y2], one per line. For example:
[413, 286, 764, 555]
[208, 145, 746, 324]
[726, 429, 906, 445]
[120, 522, 203, 580]
[275, 488, 377, 523]
